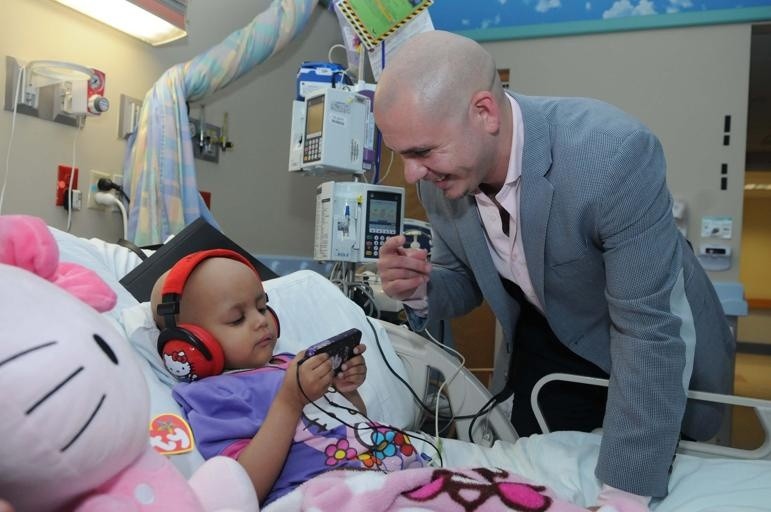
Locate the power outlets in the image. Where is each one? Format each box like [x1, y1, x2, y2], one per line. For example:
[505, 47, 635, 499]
[86, 170, 111, 212]
[55, 165, 79, 206]
[112, 176, 124, 211]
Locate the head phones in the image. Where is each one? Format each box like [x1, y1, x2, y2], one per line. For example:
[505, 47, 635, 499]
[156, 248, 280, 383]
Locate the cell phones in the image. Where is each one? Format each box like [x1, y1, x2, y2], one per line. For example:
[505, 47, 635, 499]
[305, 328, 362, 377]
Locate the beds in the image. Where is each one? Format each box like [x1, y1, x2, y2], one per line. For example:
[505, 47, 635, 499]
[0, 225, 771, 512]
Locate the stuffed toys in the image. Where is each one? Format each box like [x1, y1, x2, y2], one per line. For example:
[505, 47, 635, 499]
[0, 214, 259, 511]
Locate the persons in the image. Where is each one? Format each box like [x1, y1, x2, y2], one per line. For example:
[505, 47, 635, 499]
[149, 256, 372, 512]
[372, 30, 736, 512]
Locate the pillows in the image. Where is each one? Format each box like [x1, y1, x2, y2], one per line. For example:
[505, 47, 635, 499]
[119, 270, 419, 494]
